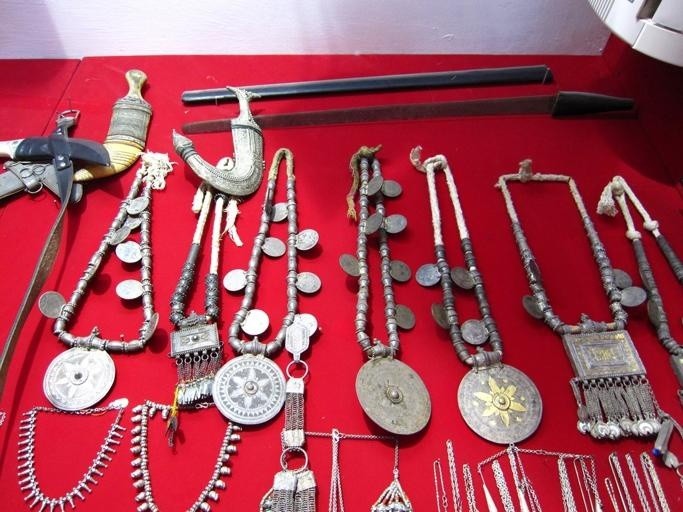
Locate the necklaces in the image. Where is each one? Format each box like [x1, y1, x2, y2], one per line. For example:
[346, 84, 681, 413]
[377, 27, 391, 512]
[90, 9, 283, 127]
[17, 144, 683, 512]
